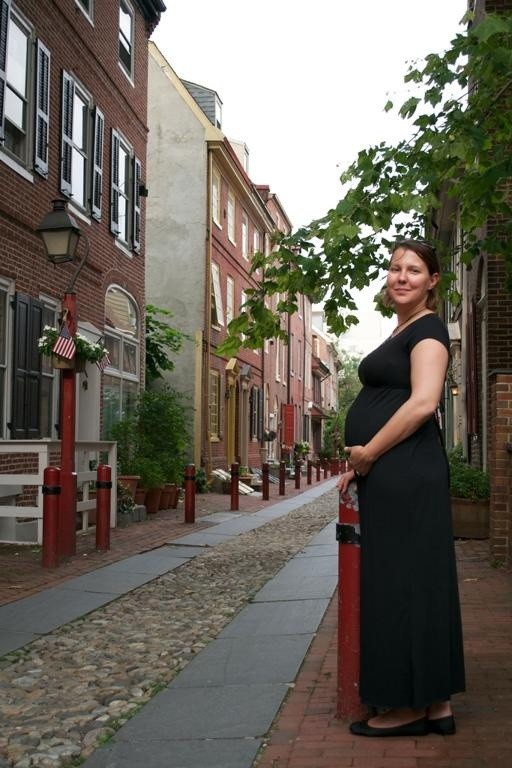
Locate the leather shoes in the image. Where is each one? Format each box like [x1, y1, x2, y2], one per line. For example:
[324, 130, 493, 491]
[348, 708, 458, 739]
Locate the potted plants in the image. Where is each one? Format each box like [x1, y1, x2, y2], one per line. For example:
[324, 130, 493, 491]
[104, 381, 187, 513]
[440, 442, 489, 538]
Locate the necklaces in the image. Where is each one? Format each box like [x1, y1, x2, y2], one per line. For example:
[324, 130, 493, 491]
[386, 307, 427, 339]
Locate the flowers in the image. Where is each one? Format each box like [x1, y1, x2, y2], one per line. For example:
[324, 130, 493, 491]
[34, 323, 111, 362]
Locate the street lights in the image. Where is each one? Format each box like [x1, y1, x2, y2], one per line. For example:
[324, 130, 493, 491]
[36, 193, 91, 562]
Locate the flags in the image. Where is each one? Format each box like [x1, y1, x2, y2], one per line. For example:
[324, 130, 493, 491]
[52, 324, 80, 363]
[98, 352, 113, 370]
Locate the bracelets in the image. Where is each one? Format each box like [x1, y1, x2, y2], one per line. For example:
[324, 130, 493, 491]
[352, 469, 359, 477]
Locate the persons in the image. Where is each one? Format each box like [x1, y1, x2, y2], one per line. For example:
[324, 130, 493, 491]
[335, 239, 463, 740]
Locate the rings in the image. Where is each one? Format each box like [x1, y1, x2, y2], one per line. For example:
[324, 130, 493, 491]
[356, 470, 361, 476]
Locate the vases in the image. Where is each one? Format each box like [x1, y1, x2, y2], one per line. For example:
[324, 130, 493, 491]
[52, 350, 87, 373]
